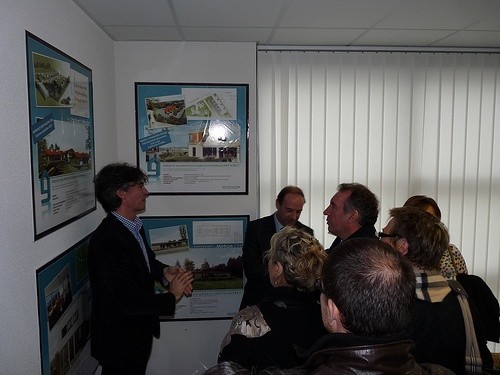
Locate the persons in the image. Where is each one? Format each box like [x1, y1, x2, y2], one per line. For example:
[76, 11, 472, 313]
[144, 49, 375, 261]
[87, 162, 194, 375]
[217, 182, 500, 375]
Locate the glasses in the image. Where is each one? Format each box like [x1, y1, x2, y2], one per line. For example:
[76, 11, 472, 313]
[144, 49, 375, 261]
[314, 277, 332, 299]
[378, 232, 402, 241]
